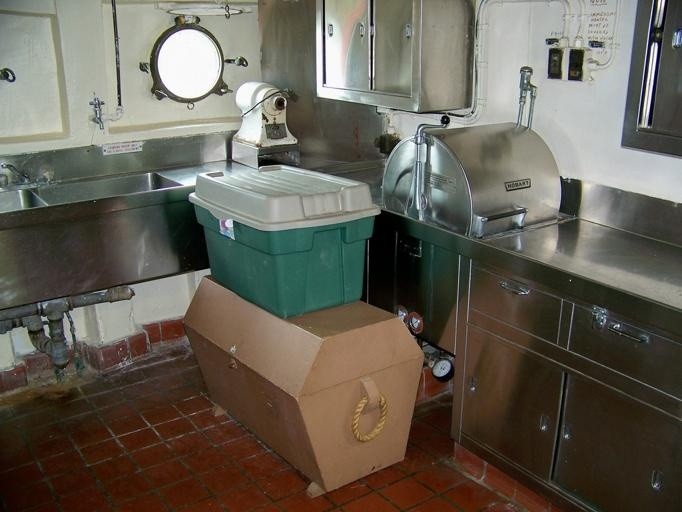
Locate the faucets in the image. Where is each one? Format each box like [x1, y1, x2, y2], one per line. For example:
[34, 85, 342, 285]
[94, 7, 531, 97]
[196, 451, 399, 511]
[2, 162, 30, 185]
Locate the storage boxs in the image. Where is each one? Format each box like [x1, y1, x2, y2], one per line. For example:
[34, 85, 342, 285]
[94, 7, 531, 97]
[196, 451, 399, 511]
[182, 165, 381, 318]
[184, 276, 426, 493]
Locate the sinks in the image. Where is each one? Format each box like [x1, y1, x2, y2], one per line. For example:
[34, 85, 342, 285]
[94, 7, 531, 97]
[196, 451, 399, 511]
[0, 190, 47, 214]
[33, 173, 184, 206]
